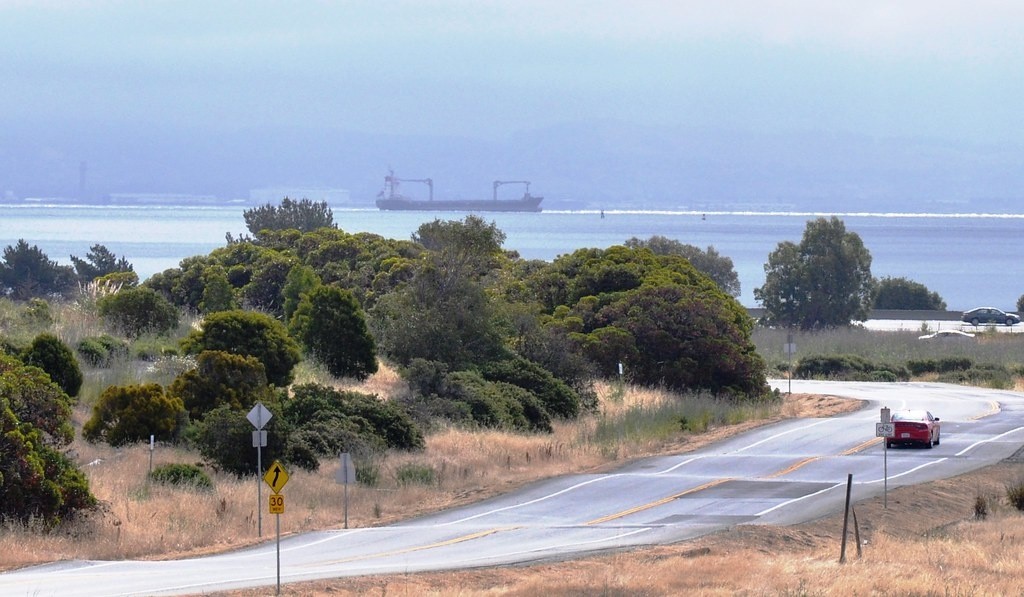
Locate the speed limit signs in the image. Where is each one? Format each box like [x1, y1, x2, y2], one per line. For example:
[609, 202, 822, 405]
[269, 494, 285, 514]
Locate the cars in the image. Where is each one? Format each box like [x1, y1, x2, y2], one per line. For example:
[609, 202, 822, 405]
[961, 307, 1022, 327]
[887, 408, 941, 449]
[918, 329, 976, 343]
